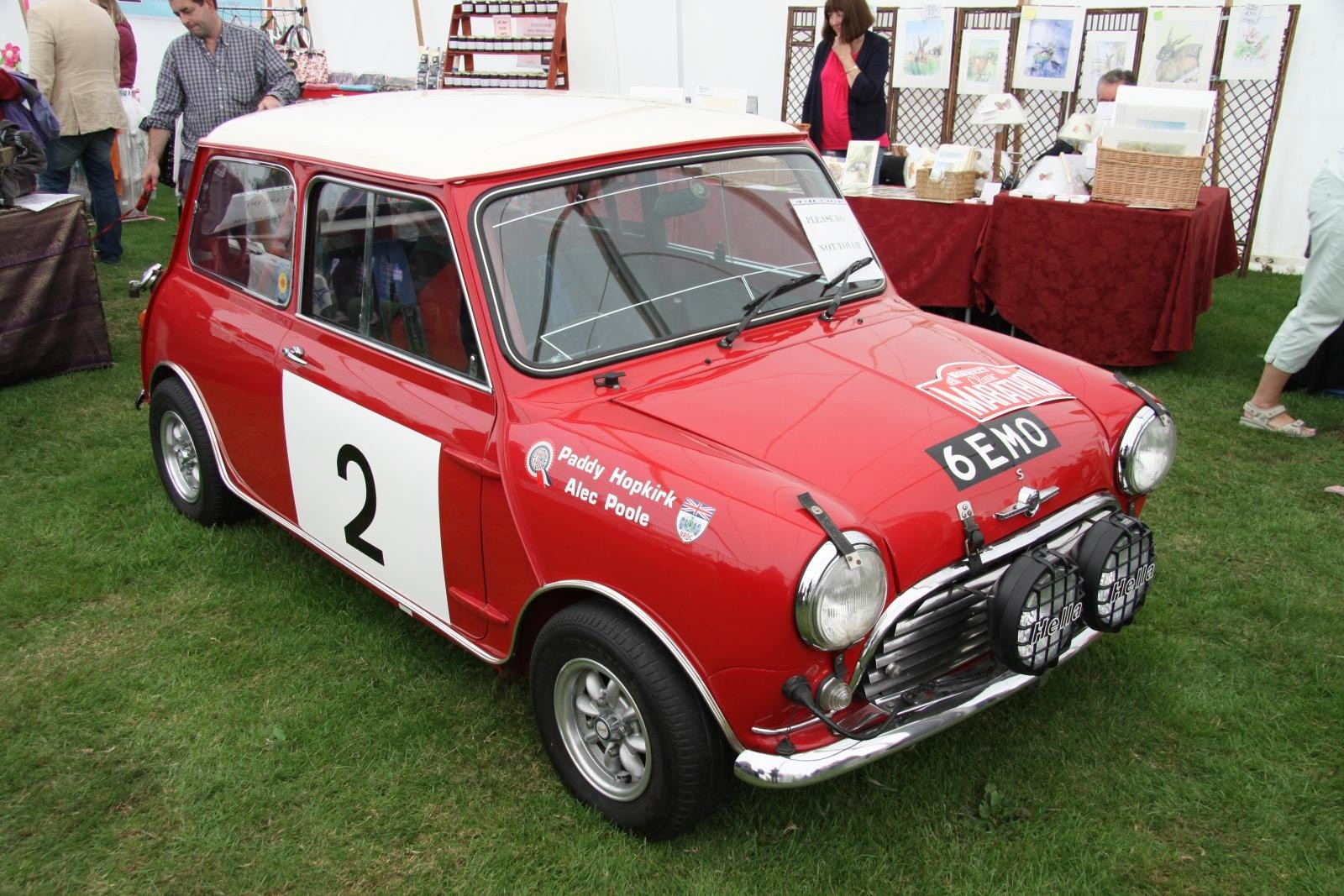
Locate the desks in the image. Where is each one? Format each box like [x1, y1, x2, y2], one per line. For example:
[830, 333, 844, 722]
[561, 171, 1240, 366]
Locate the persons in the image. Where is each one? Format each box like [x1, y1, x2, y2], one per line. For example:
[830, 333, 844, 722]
[1237, 152, 1344, 438]
[138, 0, 300, 225]
[25, 0, 156, 265]
[801, 0, 890, 186]
[1026, 67, 1137, 174]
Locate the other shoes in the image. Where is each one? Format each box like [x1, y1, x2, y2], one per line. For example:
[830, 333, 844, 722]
[92, 252, 120, 264]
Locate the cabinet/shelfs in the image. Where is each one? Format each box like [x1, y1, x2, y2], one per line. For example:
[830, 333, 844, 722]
[440, 2, 569, 90]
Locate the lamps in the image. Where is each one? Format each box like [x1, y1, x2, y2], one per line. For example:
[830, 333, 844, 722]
[1058, 113, 1093, 153]
[972, 91, 1027, 182]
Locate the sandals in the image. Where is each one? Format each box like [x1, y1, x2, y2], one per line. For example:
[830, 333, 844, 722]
[1239, 400, 1317, 437]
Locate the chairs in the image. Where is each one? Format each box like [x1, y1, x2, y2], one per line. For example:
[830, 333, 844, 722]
[325, 235, 485, 380]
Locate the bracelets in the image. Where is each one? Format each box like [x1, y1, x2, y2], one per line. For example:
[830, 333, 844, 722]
[845, 64, 858, 74]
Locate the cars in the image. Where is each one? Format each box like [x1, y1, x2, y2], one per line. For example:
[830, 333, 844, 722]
[126, 88, 1174, 847]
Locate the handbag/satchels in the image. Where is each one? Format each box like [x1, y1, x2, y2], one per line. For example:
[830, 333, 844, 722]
[274, 24, 328, 84]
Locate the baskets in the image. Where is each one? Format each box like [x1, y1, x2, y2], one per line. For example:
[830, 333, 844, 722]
[915, 169, 976, 201]
[1090, 136, 1210, 209]
